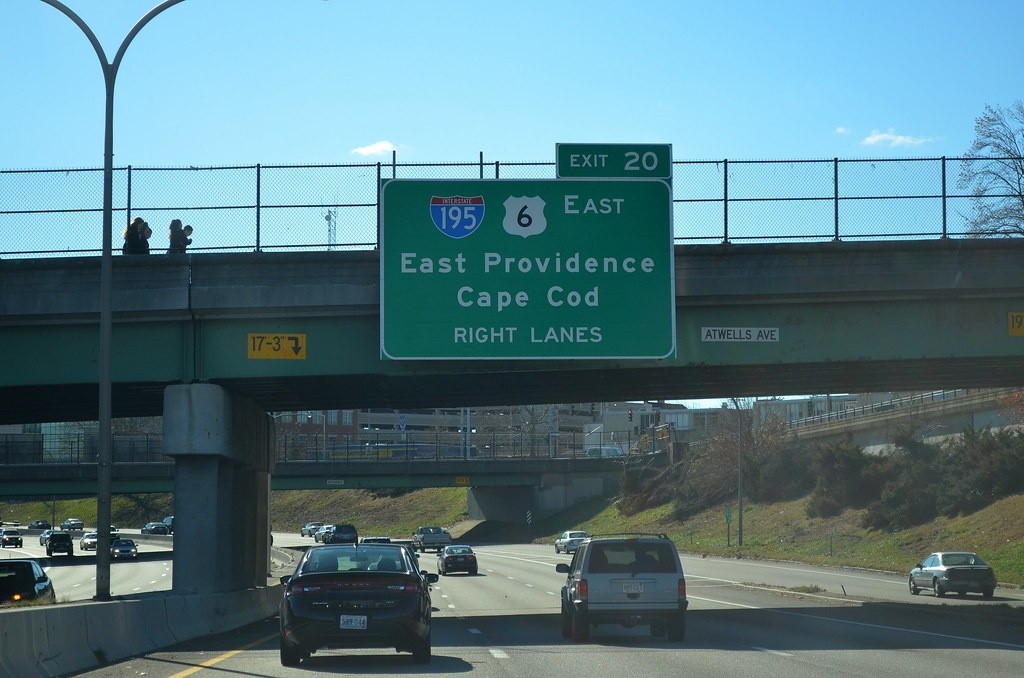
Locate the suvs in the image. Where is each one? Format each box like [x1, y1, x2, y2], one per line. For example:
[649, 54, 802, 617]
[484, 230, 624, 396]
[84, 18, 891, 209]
[163, 516, 173, 534]
[323, 524, 358, 544]
[556, 532, 689, 643]
[301, 522, 323, 537]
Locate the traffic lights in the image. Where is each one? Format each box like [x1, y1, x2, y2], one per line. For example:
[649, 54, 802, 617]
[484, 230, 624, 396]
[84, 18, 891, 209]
[656, 410, 661, 423]
[628, 409, 633, 421]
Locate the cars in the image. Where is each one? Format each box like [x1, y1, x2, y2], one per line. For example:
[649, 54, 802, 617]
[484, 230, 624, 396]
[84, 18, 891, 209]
[39, 530, 63, 546]
[909, 551, 997, 599]
[28, 520, 51, 529]
[555, 531, 588, 554]
[315, 525, 334, 542]
[141, 522, 168, 535]
[0, 560, 56, 608]
[0, 529, 23, 548]
[110, 539, 138, 560]
[279, 543, 439, 667]
[79, 532, 97, 551]
[436, 545, 478, 576]
[60, 518, 84, 530]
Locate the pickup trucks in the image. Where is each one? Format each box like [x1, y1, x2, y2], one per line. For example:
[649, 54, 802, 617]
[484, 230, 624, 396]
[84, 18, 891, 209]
[412, 526, 452, 552]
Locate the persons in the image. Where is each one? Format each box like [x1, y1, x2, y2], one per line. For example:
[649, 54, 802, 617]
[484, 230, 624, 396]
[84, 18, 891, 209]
[122, 217, 152, 255]
[165, 219, 193, 254]
